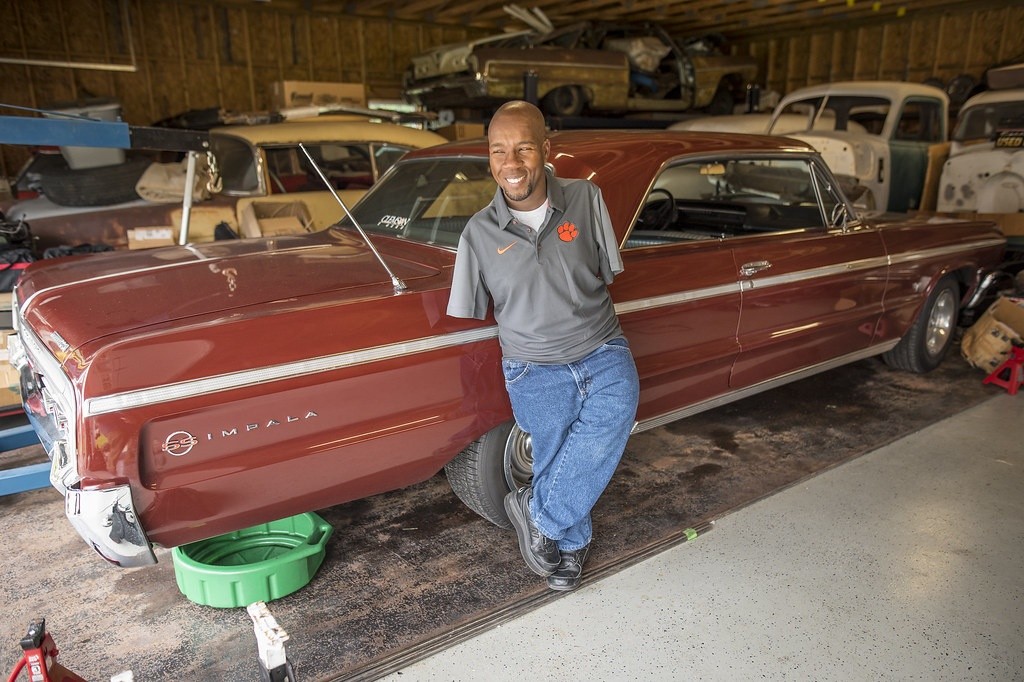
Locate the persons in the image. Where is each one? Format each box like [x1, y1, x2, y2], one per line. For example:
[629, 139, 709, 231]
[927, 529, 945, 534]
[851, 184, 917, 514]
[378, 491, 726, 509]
[446, 100, 640, 591]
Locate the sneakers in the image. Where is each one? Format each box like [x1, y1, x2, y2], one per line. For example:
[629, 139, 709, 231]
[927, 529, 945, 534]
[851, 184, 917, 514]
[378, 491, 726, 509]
[504, 486, 561, 578]
[546, 543, 590, 591]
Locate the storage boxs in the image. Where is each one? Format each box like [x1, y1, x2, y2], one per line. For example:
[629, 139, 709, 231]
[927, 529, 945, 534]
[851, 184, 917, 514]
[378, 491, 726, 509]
[436, 122, 485, 142]
[272, 80, 366, 110]
[42, 102, 124, 170]
[960, 295, 1024, 381]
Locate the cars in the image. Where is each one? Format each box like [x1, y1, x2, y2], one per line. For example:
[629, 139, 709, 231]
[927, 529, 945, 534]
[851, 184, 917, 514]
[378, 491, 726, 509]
[6, 127, 1007, 571]
[1, 103, 450, 291]
[408, 22, 1023, 221]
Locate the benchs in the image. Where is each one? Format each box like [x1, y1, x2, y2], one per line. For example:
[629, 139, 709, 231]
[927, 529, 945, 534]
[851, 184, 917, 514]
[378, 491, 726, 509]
[674, 206, 747, 235]
